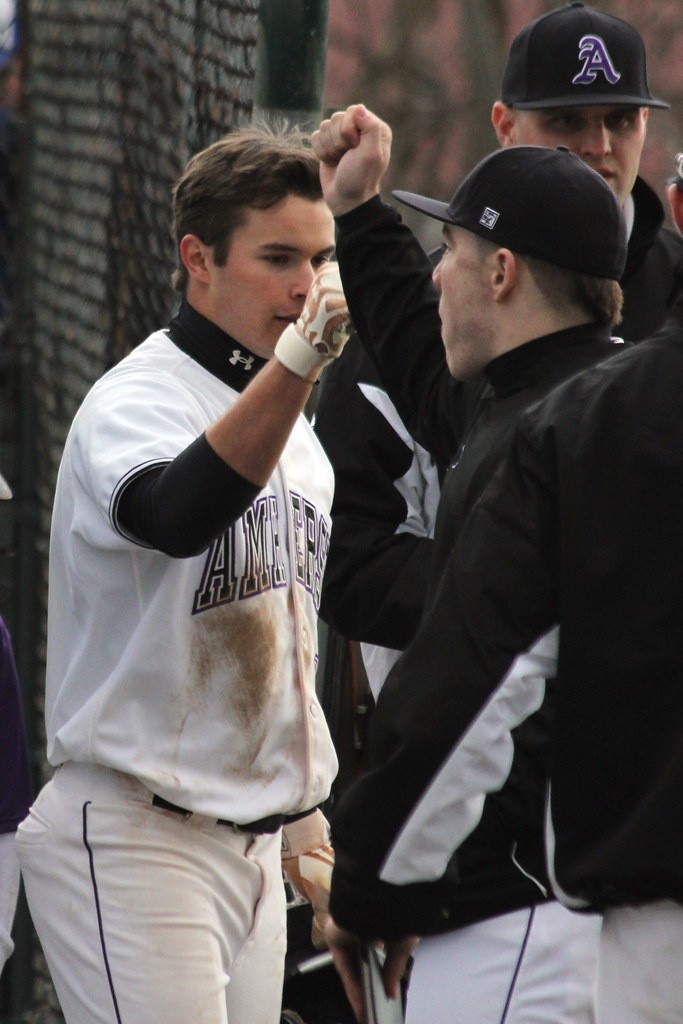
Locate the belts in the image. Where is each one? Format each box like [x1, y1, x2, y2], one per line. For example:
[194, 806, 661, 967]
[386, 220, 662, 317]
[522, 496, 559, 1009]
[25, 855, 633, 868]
[153, 793, 286, 835]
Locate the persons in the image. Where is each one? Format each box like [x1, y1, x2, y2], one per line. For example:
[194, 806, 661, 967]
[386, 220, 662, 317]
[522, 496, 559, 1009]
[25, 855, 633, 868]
[13, 125, 352, 1024]
[0, 615, 32, 1024]
[304, 2, 683, 1024]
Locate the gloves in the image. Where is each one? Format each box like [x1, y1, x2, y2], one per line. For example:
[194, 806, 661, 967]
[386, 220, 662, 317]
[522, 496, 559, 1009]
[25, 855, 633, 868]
[274, 260, 355, 383]
[279, 807, 336, 950]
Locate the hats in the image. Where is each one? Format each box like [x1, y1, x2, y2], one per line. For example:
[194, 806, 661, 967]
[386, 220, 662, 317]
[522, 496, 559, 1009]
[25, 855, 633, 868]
[502, 2, 672, 110]
[392, 144, 627, 280]
